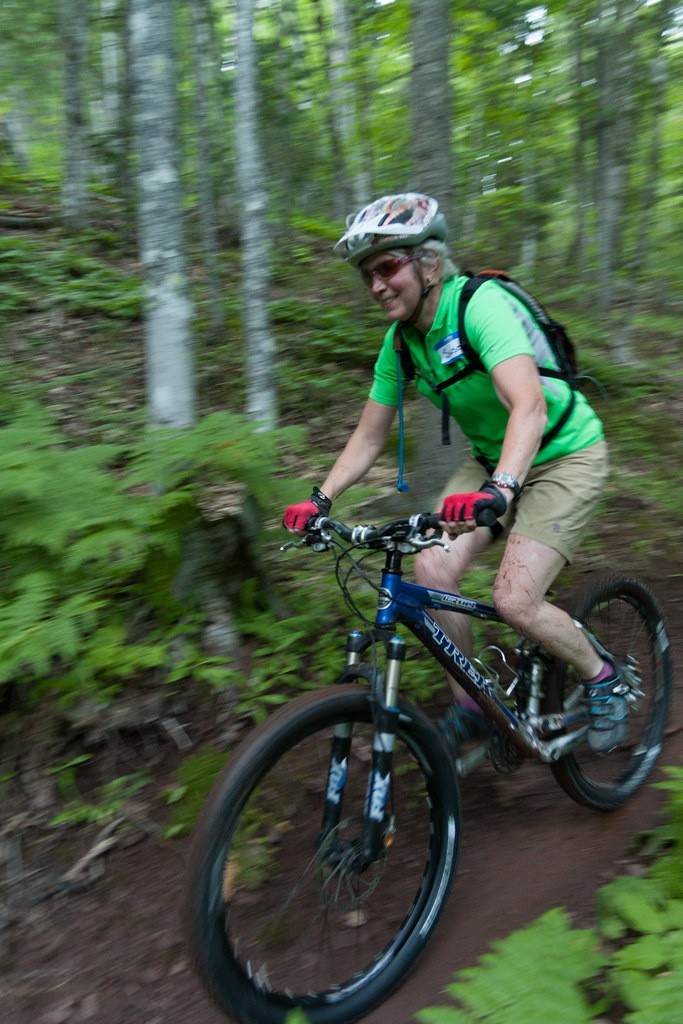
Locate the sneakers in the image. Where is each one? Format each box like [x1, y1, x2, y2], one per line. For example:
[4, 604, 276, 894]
[581, 654, 631, 754]
[437, 701, 500, 756]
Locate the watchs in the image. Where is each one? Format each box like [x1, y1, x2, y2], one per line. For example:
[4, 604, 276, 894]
[490, 473, 522, 497]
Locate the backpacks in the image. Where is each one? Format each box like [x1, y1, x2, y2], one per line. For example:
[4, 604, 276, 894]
[390, 270, 606, 454]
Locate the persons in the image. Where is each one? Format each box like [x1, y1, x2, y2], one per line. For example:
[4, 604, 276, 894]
[280, 192, 634, 750]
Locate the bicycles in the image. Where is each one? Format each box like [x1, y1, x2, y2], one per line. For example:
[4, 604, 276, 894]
[182, 489, 672, 1023]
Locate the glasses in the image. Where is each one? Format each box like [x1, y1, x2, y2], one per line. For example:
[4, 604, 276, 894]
[361, 252, 424, 287]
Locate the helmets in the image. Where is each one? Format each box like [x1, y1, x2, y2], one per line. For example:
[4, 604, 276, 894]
[333, 192, 448, 270]
